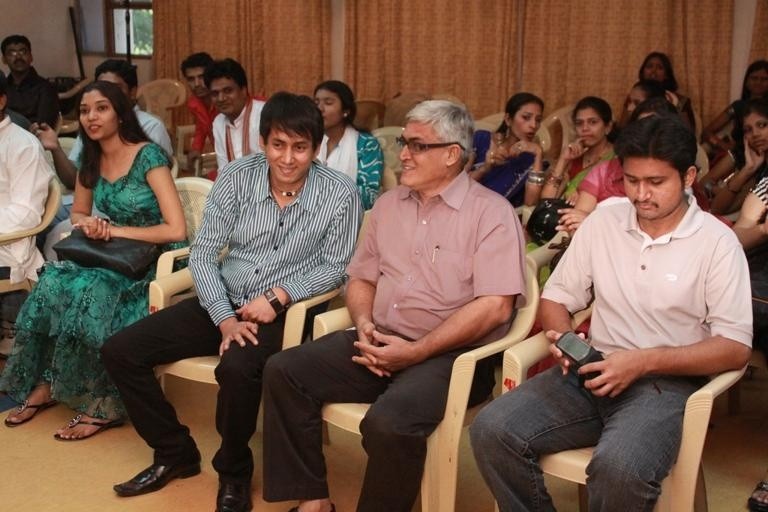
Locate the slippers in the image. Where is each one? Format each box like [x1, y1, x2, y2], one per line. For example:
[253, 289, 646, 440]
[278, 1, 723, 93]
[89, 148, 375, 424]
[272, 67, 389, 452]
[54, 413, 125, 441]
[5, 394, 59, 427]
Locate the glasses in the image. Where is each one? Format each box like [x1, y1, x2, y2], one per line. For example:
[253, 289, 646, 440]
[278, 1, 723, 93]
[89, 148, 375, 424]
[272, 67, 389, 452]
[395, 137, 458, 153]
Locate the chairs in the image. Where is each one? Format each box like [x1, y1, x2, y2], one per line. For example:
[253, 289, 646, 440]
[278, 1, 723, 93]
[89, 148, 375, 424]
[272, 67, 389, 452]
[149, 95, 416, 490]
[0, 66, 222, 406]
[313, 100, 747, 511]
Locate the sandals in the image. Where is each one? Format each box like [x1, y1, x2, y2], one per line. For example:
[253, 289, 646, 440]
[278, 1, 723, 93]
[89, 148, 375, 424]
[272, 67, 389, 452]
[746, 481, 768, 512]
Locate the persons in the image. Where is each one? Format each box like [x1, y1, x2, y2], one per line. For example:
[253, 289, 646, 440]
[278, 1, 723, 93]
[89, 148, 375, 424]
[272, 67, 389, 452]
[1, 80, 55, 348]
[261, 99, 530, 512]
[29, 55, 174, 257]
[1, 33, 59, 147]
[181, 53, 267, 182]
[199, 57, 270, 174]
[314, 81, 382, 211]
[469, 118, 753, 512]
[100, 92, 363, 512]
[1, 80, 188, 441]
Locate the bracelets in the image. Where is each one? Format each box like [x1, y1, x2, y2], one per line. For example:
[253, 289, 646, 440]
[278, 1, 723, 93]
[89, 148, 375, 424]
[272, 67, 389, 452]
[264, 288, 285, 317]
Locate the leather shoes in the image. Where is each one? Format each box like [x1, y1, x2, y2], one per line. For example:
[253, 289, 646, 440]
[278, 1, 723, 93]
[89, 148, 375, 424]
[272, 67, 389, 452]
[216, 481, 253, 511]
[112, 456, 202, 496]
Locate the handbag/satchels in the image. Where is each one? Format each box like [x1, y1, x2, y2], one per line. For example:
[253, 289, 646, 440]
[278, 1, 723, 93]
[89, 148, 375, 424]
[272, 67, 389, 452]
[527, 198, 575, 245]
[51, 220, 160, 279]
